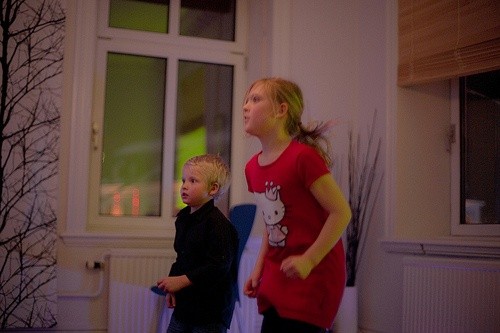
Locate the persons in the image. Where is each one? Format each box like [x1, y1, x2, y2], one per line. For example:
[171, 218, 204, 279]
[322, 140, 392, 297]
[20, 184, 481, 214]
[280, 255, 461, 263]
[242, 76, 353, 333]
[156, 154, 240, 333]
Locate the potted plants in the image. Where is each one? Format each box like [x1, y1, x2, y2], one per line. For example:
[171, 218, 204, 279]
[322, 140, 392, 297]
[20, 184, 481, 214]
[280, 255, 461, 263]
[314, 107, 388, 333]
